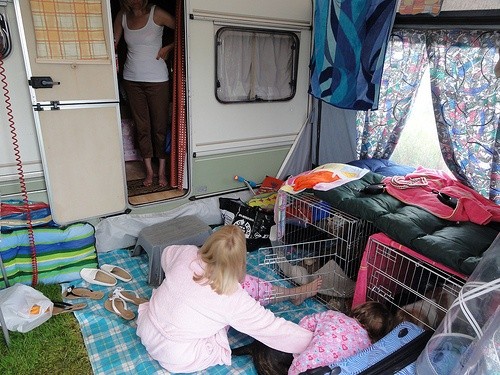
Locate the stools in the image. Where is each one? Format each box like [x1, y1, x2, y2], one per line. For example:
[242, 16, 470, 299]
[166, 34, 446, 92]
[132, 215, 213, 286]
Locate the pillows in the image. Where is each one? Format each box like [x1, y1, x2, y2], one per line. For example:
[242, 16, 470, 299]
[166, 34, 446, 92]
[0, 222, 99, 288]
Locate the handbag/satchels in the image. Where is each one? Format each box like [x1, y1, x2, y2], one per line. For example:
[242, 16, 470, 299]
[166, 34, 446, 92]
[0, 284, 54, 334]
[219, 196, 273, 253]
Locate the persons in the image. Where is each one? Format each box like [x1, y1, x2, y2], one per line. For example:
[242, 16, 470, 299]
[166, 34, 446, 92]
[112, 0, 176, 187]
[288, 302, 389, 375]
[136, 225, 314, 373]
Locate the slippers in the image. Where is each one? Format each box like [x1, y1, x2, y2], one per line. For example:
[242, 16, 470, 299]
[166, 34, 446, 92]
[101, 263, 132, 282]
[105, 297, 136, 320]
[80, 267, 118, 286]
[51, 301, 87, 316]
[109, 288, 150, 306]
[62, 287, 104, 299]
